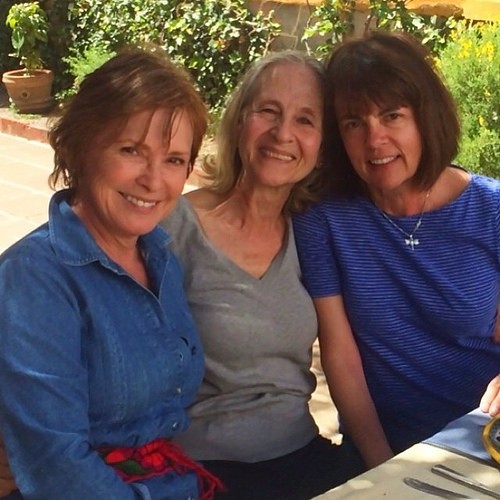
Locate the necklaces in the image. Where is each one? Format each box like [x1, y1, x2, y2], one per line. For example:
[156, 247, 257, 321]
[361, 184, 431, 251]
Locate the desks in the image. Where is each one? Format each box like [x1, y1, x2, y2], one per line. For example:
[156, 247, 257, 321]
[302, 407, 500, 500]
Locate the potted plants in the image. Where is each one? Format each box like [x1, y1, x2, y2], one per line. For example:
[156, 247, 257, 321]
[2, 1, 55, 113]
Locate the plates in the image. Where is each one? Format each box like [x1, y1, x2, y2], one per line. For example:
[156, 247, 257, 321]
[482, 411, 500, 468]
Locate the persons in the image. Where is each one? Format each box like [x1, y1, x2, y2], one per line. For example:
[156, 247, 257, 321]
[1, 50, 344, 500]
[292, 32, 500, 469]
[1, 49, 224, 500]
